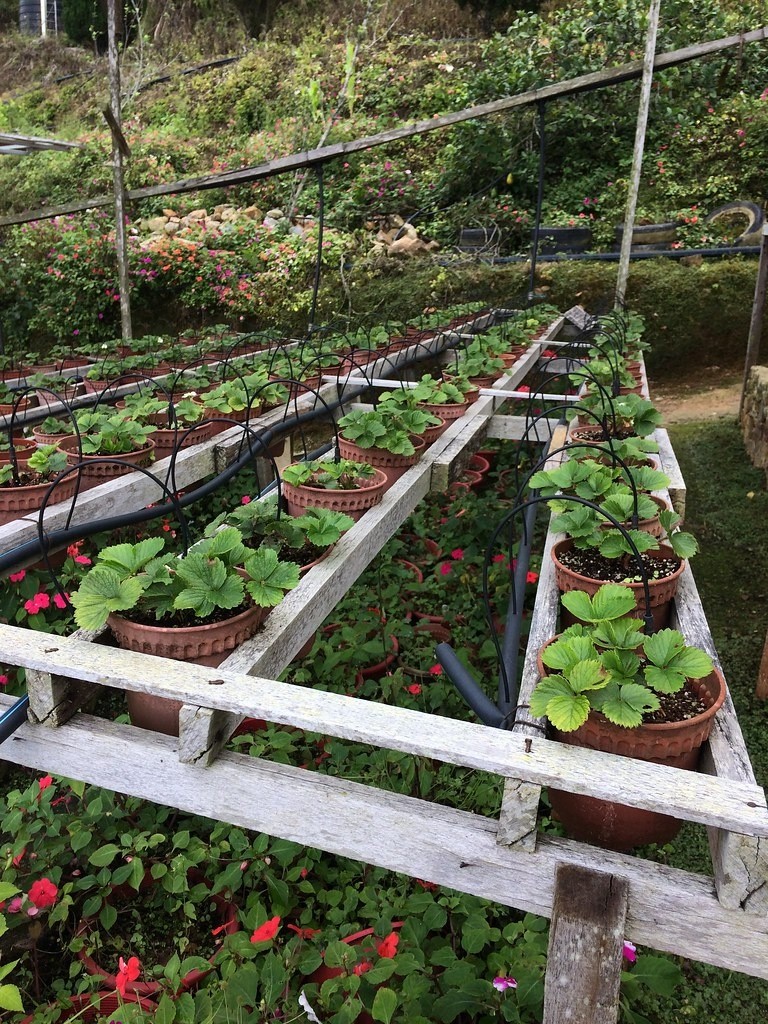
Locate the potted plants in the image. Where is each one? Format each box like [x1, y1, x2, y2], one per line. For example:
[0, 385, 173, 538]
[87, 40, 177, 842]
[0, 301, 727, 1024]
[612, 205, 686, 252]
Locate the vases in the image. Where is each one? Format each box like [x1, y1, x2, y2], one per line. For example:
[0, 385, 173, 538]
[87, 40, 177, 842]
[532, 225, 593, 259]
[457, 228, 510, 247]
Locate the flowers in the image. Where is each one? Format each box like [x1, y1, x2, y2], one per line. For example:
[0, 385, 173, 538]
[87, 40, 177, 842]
[468, 191, 531, 227]
[541, 206, 594, 228]
[348, 153, 433, 215]
